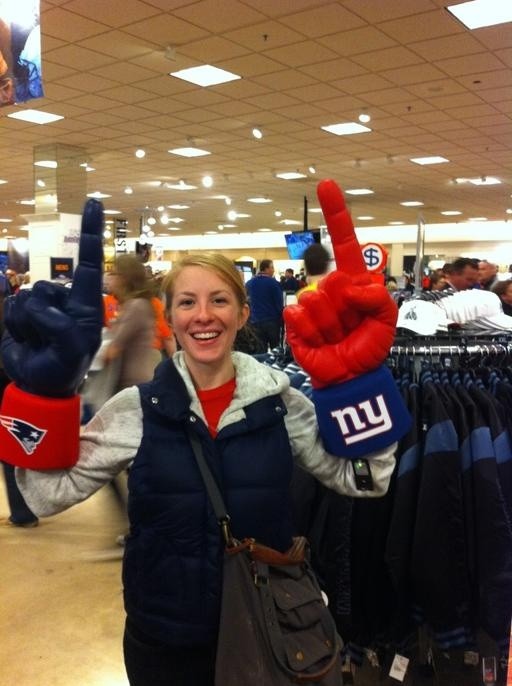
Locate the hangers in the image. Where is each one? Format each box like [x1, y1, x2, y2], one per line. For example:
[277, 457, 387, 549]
[388, 330, 512, 383]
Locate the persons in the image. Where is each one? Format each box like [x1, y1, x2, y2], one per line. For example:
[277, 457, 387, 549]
[81, 255, 182, 517]
[242, 242, 330, 355]
[12, 250, 399, 686]
[1, 270, 40, 527]
[390, 258, 511, 317]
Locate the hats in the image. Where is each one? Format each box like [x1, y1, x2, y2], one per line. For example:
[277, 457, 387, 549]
[398, 286, 511, 337]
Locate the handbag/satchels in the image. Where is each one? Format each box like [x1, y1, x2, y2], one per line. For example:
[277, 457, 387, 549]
[215, 533, 342, 686]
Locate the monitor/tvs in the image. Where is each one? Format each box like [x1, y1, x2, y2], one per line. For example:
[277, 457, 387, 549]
[284, 231, 316, 260]
